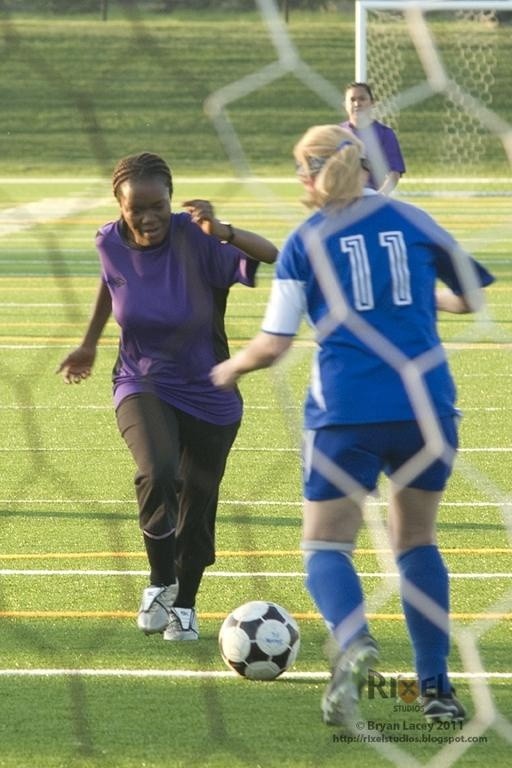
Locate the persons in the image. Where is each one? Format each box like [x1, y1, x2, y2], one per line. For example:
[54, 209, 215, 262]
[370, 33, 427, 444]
[56, 153, 279, 641]
[210, 125, 495, 724]
[339, 82, 406, 196]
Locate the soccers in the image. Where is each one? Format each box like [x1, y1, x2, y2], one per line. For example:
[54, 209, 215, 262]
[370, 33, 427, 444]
[217, 600, 301, 682]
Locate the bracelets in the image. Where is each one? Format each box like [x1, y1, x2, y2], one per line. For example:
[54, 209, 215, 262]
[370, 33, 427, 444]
[219, 222, 234, 246]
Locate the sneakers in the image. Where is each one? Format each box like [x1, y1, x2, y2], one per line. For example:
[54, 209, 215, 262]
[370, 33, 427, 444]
[419, 693, 470, 725]
[320, 636, 380, 728]
[163, 608, 200, 642]
[135, 581, 180, 636]
